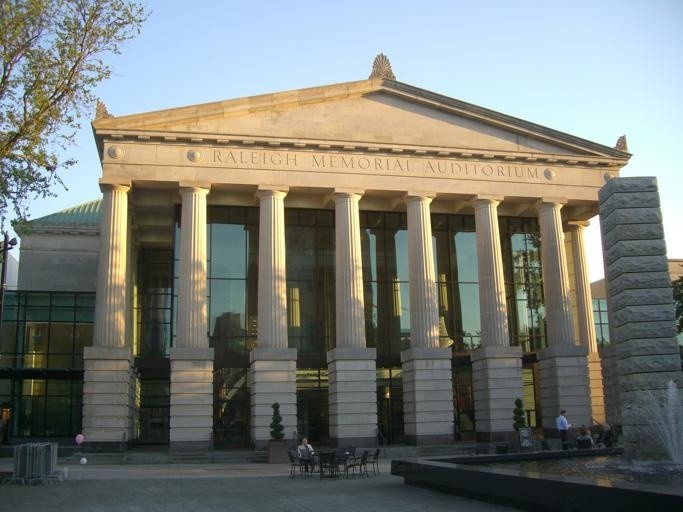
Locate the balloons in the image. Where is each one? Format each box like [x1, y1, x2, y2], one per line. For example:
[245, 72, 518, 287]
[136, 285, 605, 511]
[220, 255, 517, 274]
[74, 433, 83, 445]
[78, 456, 87, 465]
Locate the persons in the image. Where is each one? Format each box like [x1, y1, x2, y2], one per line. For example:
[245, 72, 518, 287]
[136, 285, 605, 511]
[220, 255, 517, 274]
[556, 409, 573, 449]
[574, 423, 595, 447]
[296, 437, 316, 473]
[592, 414, 613, 449]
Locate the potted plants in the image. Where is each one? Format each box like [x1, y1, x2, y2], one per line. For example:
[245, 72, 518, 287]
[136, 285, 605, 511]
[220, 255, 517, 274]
[510, 398, 527, 448]
[267, 402, 290, 464]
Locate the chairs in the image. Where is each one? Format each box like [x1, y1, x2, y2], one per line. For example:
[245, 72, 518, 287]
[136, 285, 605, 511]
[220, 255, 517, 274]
[287, 445, 381, 479]
[576, 439, 592, 449]
